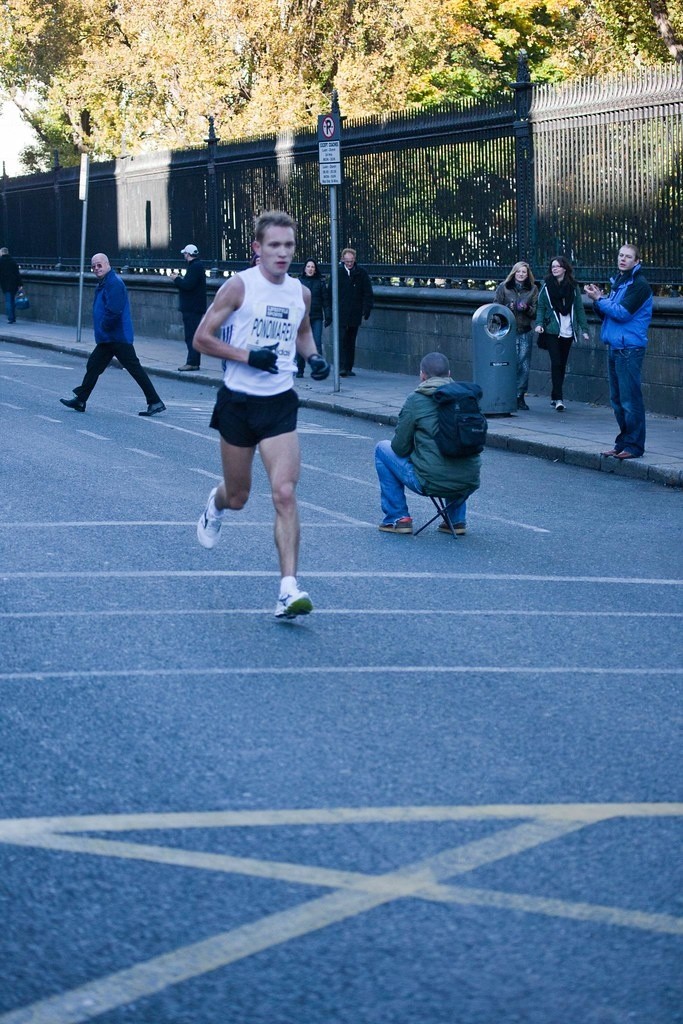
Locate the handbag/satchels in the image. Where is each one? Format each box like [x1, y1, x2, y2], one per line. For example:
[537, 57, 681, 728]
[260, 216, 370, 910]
[15, 290, 29, 310]
[536, 334, 546, 349]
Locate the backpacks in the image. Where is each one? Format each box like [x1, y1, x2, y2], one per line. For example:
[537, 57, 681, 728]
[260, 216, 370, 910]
[433, 378, 488, 459]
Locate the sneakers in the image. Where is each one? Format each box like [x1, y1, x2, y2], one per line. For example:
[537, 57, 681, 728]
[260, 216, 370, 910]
[275, 588, 312, 619]
[197, 487, 224, 549]
[438, 521, 466, 534]
[379, 517, 412, 533]
[555, 400, 564, 410]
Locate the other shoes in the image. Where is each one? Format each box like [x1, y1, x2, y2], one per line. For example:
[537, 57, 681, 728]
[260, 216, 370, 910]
[613, 450, 634, 458]
[340, 369, 348, 376]
[348, 371, 355, 376]
[602, 448, 618, 456]
[296, 372, 302, 377]
[178, 364, 199, 371]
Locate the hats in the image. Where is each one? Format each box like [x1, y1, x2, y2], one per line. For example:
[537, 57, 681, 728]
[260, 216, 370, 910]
[181, 244, 198, 254]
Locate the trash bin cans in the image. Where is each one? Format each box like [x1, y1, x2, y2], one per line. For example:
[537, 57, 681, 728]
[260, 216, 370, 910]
[470, 302, 520, 416]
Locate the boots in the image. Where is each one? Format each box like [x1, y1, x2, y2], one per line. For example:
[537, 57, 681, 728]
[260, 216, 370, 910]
[518, 397, 529, 410]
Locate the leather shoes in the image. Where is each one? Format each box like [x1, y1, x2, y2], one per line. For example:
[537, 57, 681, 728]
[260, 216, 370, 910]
[60, 396, 86, 412]
[139, 401, 166, 416]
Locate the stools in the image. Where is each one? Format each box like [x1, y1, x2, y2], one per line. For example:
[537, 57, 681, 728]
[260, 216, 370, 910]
[414, 489, 472, 539]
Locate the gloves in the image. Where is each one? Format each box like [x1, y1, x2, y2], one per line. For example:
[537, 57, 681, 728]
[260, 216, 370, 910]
[518, 303, 527, 311]
[308, 354, 329, 380]
[249, 349, 279, 374]
[363, 309, 371, 320]
[324, 318, 331, 327]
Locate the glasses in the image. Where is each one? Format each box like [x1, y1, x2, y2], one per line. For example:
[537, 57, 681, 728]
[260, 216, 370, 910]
[92, 262, 106, 270]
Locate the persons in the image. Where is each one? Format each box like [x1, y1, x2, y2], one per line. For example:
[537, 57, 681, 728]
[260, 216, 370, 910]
[584, 244, 652, 459]
[192, 210, 330, 620]
[168, 243, 207, 371]
[328, 248, 373, 376]
[296, 259, 331, 379]
[374, 352, 482, 534]
[533, 255, 590, 411]
[59, 253, 167, 416]
[493, 260, 538, 410]
[250, 253, 261, 267]
[0, 247, 23, 324]
